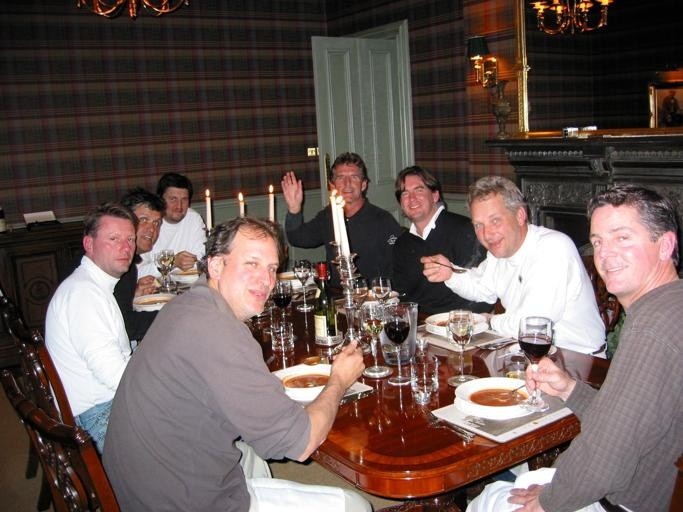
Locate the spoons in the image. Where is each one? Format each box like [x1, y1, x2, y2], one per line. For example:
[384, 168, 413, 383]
[433, 261, 469, 274]
[497, 349, 526, 359]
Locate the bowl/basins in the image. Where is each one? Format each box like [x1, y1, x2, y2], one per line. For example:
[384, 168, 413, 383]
[277, 270, 316, 290]
[274, 362, 340, 408]
[454, 377, 537, 412]
[169, 269, 200, 284]
[424, 311, 487, 332]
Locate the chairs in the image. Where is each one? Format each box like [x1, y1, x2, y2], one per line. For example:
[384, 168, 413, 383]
[0, 288, 77, 512]
[669, 449, 683, 512]
[0, 371, 122, 512]
[493, 254, 622, 334]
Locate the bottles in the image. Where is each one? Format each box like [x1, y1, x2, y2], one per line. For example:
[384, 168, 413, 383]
[313, 261, 343, 349]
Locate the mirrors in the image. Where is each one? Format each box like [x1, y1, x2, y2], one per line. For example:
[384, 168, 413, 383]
[519, 0, 683, 132]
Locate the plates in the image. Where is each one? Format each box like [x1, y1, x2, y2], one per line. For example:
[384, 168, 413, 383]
[453, 395, 540, 420]
[508, 342, 559, 358]
[425, 323, 488, 338]
[132, 293, 176, 310]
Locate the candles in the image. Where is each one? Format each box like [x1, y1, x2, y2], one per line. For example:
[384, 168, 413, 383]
[338, 207, 350, 257]
[268, 194, 274, 222]
[206, 195, 212, 231]
[335, 202, 342, 256]
[328, 196, 339, 244]
[240, 201, 246, 217]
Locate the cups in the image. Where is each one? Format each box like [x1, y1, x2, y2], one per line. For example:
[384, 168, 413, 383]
[360, 308, 394, 379]
[272, 279, 294, 320]
[161, 249, 176, 289]
[447, 309, 481, 388]
[382, 305, 412, 387]
[409, 355, 433, 405]
[518, 317, 555, 414]
[372, 276, 392, 308]
[270, 322, 294, 353]
[410, 356, 439, 394]
[353, 277, 368, 308]
[380, 301, 419, 367]
[294, 258, 315, 312]
[154, 252, 174, 292]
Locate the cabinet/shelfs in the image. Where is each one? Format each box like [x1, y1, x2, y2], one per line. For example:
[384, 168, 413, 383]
[0, 220, 87, 368]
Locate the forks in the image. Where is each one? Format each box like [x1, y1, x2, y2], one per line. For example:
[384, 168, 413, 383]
[425, 416, 475, 444]
[420, 406, 478, 438]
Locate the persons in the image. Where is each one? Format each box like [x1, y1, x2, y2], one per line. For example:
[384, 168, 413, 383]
[464, 184, 683, 512]
[419, 176, 607, 357]
[370, 165, 494, 313]
[100, 217, 373, 512]
[43, 202, 131, 451]
[112, 189, 163, 341]
[134, 171, 207, 283]
[280, 152, 400, 290]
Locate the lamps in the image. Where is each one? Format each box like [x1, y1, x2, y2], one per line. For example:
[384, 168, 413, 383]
[529, 0, 615, 35]
[78, 0, 190, 19]
[466, 35, 490, 84]
